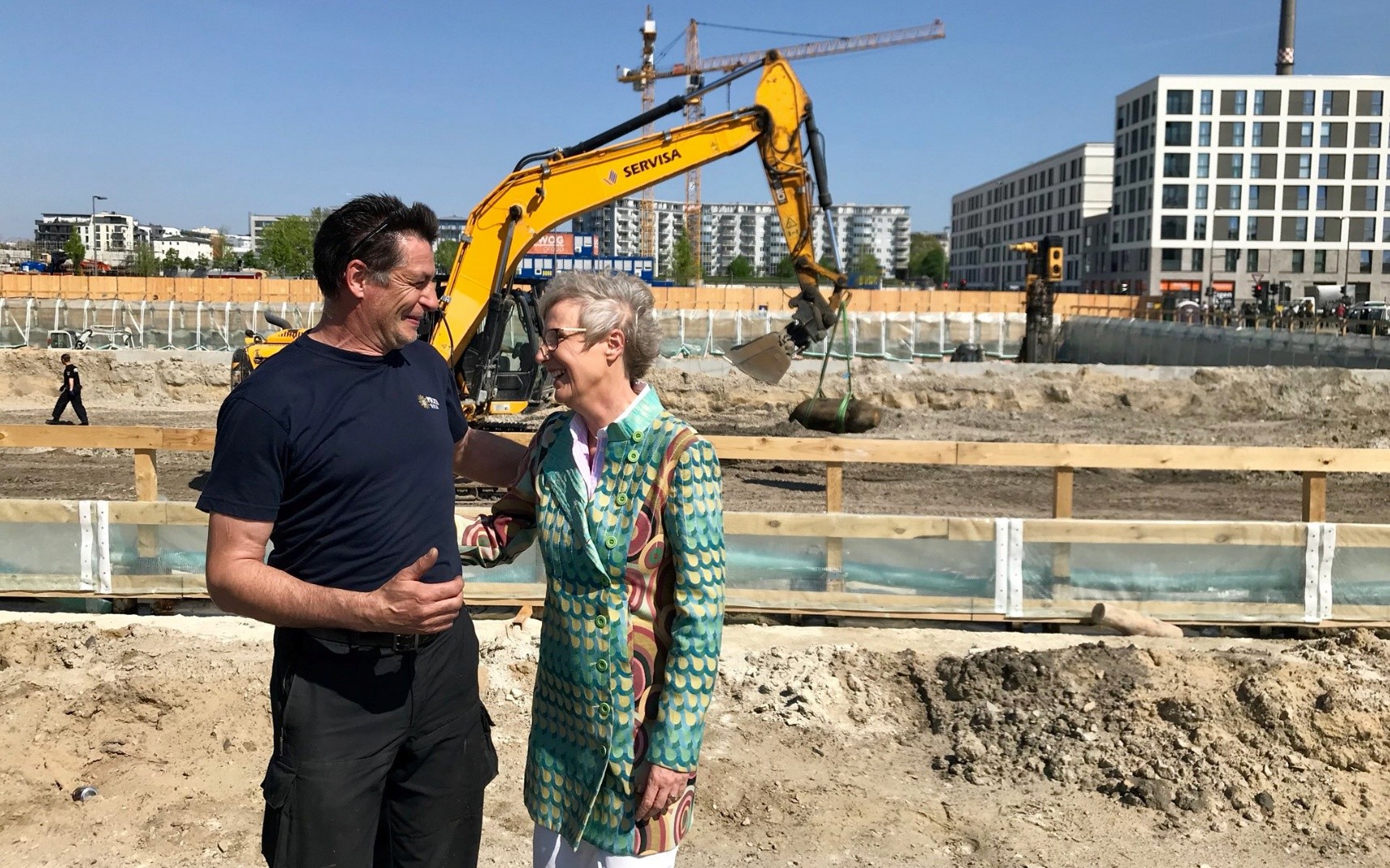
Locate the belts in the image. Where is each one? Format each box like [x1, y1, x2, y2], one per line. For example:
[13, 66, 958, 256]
[305, 624, 438, 652]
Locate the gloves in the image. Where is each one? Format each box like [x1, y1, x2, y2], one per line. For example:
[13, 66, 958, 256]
[60, 386, 64, 391]
[69, 391, 74, 398]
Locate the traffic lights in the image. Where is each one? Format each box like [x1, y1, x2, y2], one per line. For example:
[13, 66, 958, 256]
[1252, 285, 1265, 299]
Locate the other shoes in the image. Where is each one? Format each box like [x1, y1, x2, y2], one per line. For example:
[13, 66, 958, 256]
[45, 418, 60, 424]
[80, 422, 88, 425]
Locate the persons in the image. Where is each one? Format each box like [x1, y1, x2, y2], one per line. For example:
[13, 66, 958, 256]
[45, 354, 88, 426]
[449, 272, 724, 868]
[197, 194, 529, 868]
[1198, 300, 1345, 330]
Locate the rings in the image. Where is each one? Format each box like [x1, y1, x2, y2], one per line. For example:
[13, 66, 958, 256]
[670, 795, 677, 801]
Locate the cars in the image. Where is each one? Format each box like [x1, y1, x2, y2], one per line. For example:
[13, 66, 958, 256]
[1281, 295, 1390, 336]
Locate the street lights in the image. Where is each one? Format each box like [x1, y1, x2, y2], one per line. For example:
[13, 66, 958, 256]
[92, 195, 109, 277]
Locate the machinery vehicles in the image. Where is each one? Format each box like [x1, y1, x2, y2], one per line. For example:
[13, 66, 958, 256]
[48, 323, 137, 352]
[224, 45, 853, 428]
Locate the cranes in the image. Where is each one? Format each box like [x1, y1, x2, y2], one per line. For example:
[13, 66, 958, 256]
[614, 7, 950, 262]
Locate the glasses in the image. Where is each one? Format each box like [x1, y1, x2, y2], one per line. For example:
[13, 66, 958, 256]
[537, 328, 629, 352]
